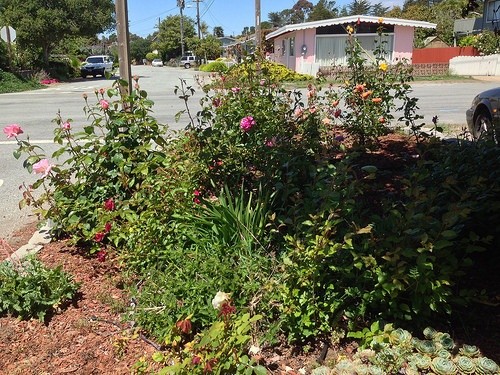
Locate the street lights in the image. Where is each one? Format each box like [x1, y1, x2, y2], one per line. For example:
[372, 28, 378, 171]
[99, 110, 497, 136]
[185, 4, 201, 39]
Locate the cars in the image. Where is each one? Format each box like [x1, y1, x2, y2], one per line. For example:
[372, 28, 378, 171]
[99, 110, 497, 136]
[151, 59, 163, 67]
[465, 86, 500, 147]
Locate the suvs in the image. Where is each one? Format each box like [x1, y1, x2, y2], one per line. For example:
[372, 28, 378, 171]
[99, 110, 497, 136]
[179, 55, 196, 69]
[81, 55, 114, 79]
